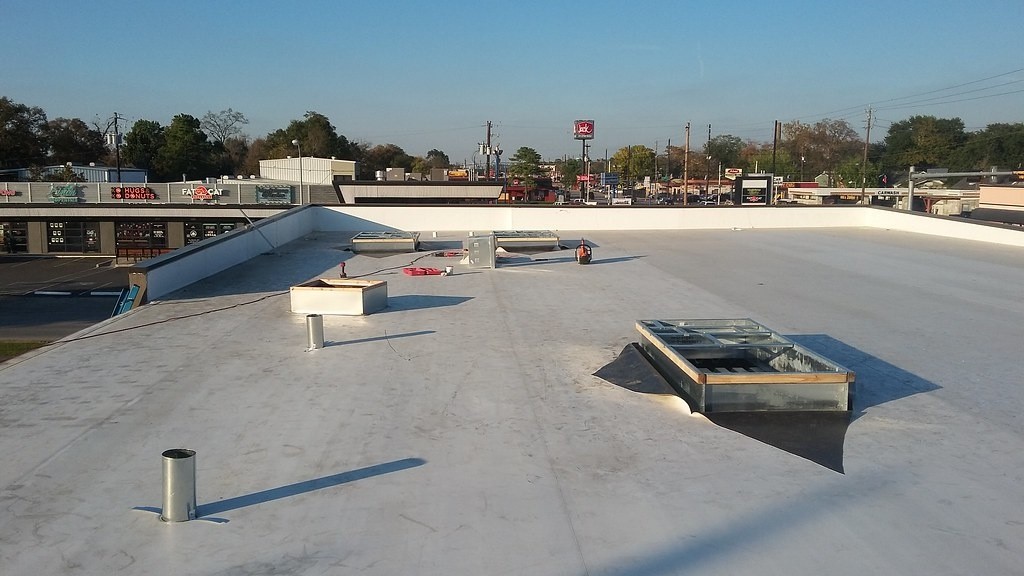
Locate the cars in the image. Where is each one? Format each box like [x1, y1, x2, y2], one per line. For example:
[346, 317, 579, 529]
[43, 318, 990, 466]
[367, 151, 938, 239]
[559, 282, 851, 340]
[677, 194, 699, 203]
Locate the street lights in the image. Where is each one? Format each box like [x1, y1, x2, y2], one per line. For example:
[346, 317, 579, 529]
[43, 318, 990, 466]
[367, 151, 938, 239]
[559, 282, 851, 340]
[292, 139, 303, 206]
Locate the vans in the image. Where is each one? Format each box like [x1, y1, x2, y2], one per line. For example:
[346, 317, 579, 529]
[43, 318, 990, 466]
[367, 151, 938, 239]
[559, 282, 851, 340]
[707, 194, 730, 202]
